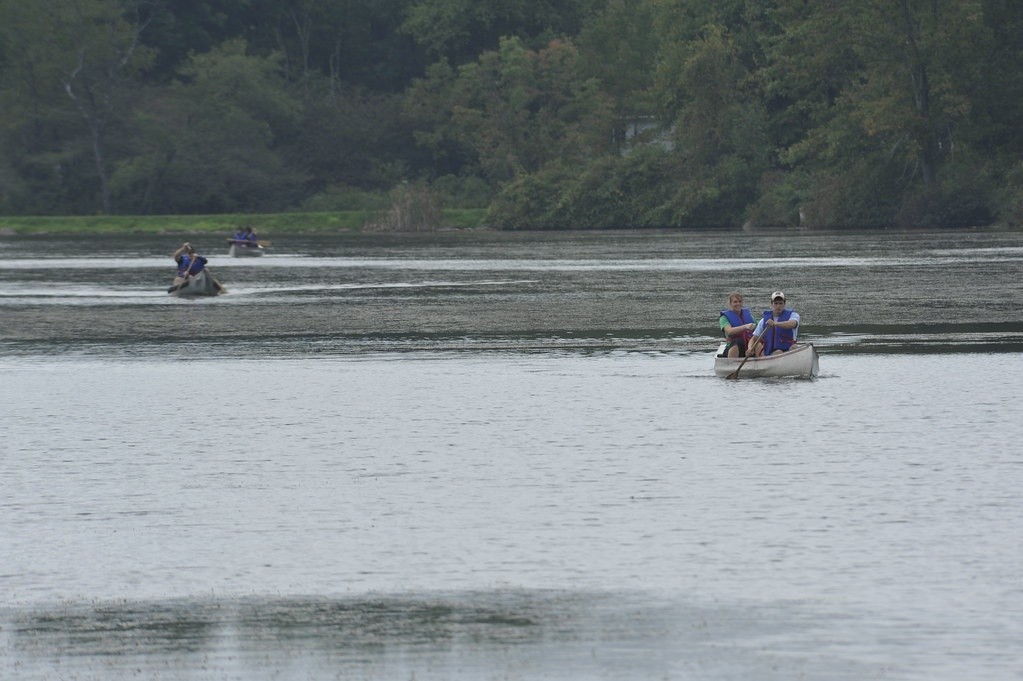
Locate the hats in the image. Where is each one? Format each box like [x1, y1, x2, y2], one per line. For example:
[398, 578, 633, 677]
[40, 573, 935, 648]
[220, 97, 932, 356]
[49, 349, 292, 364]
[770, 291, 786, 304]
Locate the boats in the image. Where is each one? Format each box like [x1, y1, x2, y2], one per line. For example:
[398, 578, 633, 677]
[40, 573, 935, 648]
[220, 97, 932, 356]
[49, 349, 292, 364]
[168, 268, 222, 298]
[713, 341, 819, 381]
[227, 237, 265, 258]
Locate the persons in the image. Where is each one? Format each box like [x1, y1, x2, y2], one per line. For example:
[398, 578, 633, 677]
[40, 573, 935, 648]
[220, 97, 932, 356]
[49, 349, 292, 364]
[718, 293, 757, 358]
[174, 242, 208, 286]
[745, 290, 800, 358]
[234, 226, 257, 247]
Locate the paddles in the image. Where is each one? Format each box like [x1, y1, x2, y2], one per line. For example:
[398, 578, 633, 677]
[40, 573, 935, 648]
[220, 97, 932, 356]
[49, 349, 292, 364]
[174, 254, 197, 294]
[189, 246, 229, 293]
[726, 322, 771, 379]
[227, 238, 272, 247]
